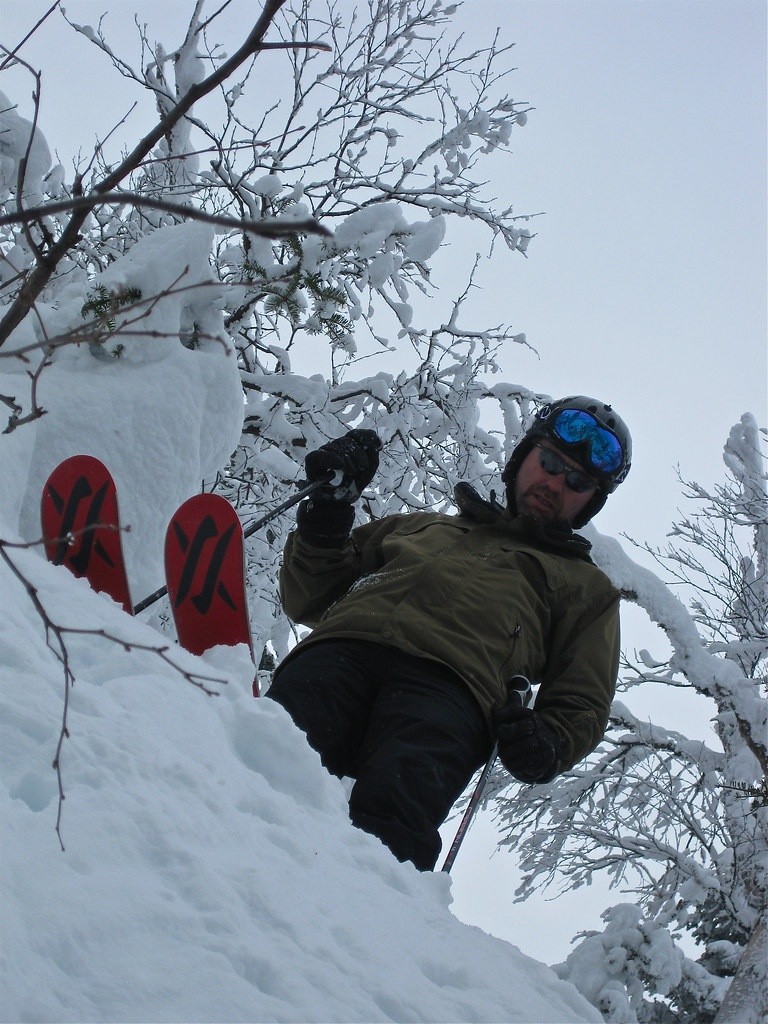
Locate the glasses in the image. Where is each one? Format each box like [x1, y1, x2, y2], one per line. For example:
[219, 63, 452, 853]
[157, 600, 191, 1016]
[546, 406, 624, 477]
[532, 441, 601, 493]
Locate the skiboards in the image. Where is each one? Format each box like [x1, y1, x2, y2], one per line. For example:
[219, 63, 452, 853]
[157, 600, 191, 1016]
[163, 495, 261, 700]
[38, 449, 131, 614]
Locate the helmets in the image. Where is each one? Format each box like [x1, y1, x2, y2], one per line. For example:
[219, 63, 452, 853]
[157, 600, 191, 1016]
[531, 395, 631, 492]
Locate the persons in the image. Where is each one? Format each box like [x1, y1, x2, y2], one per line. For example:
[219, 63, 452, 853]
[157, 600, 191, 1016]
[263, 395, 632, 874]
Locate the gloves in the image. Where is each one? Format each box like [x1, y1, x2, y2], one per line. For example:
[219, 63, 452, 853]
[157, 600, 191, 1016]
[305, 429, 383, 521]
[494, 707, 561, 785]
